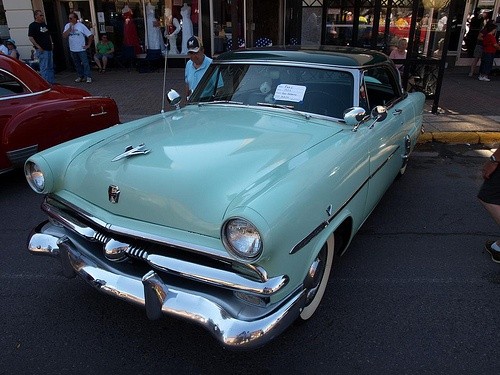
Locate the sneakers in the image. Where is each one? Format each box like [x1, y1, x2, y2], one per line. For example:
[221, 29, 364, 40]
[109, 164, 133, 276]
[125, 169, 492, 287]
[485, 239, 500, 263]
[86, 78, 92, 83]
[74, 76, 82, 83]
[478, 74, 490, 81]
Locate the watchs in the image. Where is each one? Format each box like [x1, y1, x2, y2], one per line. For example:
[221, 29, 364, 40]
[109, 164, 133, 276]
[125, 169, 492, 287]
[489, 156, 500, 166]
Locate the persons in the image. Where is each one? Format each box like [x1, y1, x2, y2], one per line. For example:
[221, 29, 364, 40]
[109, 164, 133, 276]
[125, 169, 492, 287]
[476, 146, 500, 263]
[330, 7, 499, 92]
[28, 9, 56, 85]
[180, 3, 194, 53]
[0, 38, 19, 59]
[184, 35, 225, 103]
[121, 5, 141, 54]
[145, 2, 164, 49]
[61, 12, 94, 84]
[94, 32, 114, 74]
[163, 8, 181, 55]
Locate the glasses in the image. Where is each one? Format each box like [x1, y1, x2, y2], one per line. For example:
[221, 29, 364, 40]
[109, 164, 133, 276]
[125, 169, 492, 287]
[187, 51, 201, 54]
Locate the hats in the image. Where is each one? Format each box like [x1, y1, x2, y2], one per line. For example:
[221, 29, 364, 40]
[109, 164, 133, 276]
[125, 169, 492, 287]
[3, 39, 16, 48]
[186, 35, 203, 52]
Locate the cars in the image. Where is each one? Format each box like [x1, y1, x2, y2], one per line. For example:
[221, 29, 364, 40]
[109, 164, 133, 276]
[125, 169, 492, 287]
[22, 44, 427, 353]
[0, 51, 121, 176]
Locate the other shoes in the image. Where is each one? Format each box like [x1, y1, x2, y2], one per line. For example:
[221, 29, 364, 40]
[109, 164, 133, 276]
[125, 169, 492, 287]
[51, 82, 60, 85]
[468, 72, 474, 76]
[102, 69, 106, 73]
[99, 68, 102, 72]
